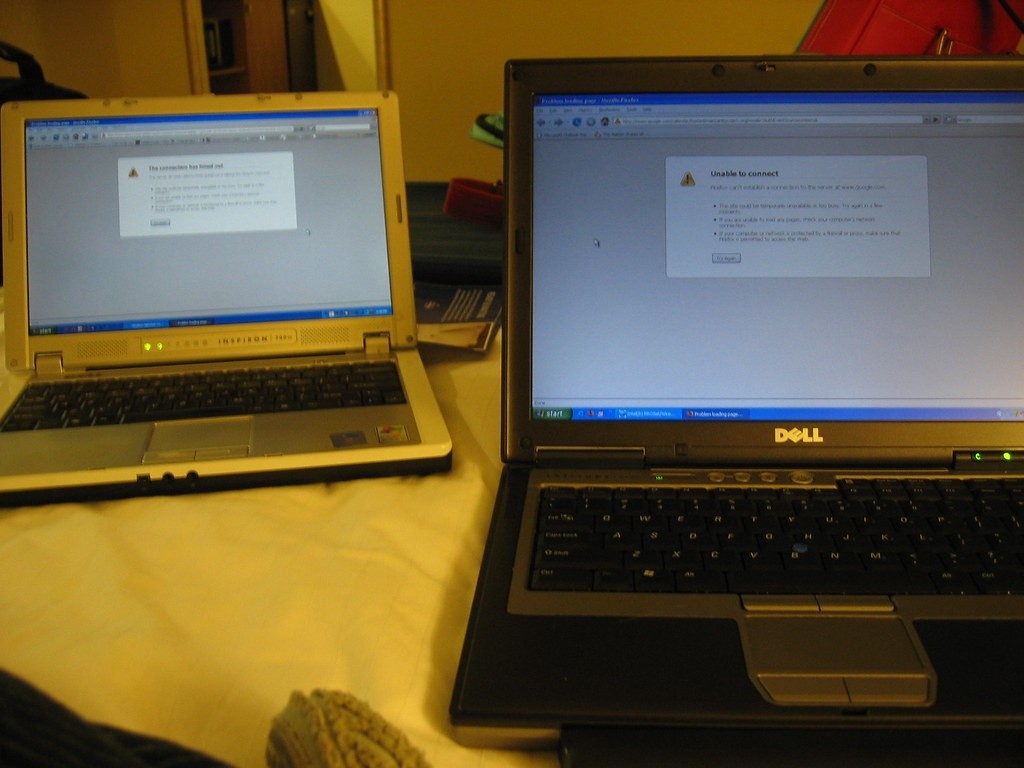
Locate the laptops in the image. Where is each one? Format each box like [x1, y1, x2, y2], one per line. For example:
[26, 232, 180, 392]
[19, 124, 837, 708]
[0, 89, 454, 508]
[449, 57, 1024, 735]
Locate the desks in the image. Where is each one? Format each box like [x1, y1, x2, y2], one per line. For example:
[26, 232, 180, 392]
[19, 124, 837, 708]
[0, 328, 561, 767]
